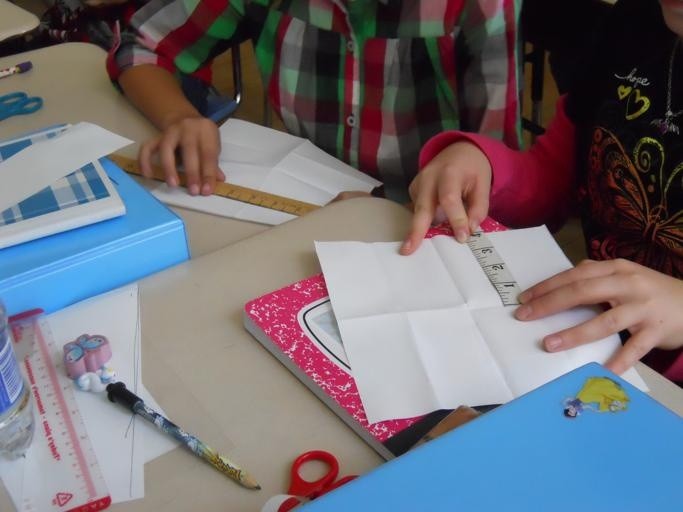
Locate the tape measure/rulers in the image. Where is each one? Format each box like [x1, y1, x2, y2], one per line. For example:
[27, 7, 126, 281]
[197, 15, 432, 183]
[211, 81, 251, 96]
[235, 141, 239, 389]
[465, 223, 524, 306]
[7, 308, 112, 512]
[106, 154, 323, 217]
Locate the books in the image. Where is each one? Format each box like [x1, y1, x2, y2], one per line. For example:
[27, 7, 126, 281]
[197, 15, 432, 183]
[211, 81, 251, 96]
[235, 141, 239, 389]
[0, 120, 127, 251]
[241, 268, 502, 464]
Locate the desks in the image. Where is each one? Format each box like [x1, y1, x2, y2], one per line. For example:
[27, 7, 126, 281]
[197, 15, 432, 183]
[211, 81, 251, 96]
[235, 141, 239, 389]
[1, 194, 683, 510]
[1, 1, 552, 260]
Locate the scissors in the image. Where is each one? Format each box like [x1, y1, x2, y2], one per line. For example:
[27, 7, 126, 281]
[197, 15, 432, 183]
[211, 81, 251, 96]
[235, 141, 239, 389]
[0, 92, 43, 122]
[276, 450, 360, 512]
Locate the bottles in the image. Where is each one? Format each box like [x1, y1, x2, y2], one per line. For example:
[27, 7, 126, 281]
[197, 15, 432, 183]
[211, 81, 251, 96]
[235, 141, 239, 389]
[0, 302, 34, 462]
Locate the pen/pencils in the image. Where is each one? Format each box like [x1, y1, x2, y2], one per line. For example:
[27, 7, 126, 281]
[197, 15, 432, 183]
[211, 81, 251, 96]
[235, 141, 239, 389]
[106, 382, 262, 489]
[0, 61, 33, 78]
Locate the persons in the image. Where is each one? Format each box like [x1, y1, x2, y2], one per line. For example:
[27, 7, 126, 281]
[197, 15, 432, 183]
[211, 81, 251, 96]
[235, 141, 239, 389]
[397, 0, 683, 379]
[0, 0, 213, 121]
[104, 0, 529, 228]
